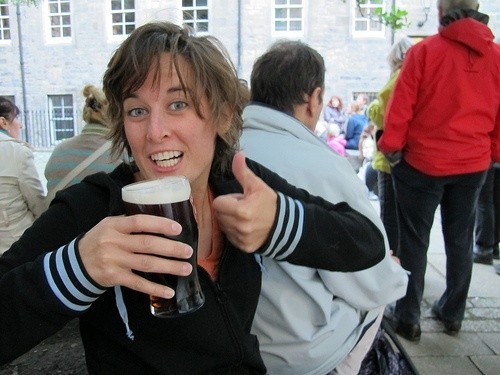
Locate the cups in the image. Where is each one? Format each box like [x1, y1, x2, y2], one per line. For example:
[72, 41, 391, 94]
[121, 175, 204, 317]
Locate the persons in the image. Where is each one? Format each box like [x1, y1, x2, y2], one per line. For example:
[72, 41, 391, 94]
[44, 85, 135, 210]
[233, 41, 413, 375]
[376, 0, 500, 342]
[0, 21, 386, 375]
[368, 39, 414, 258]
[472, 163, 500, 265]
[314, 93, 378, 200]
[0, 96, 47, 258]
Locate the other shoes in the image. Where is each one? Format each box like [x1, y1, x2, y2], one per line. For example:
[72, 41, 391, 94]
[473, 246, 500, 265]
[431, 299, 461, 335]
[383, 304, 421, 342]
